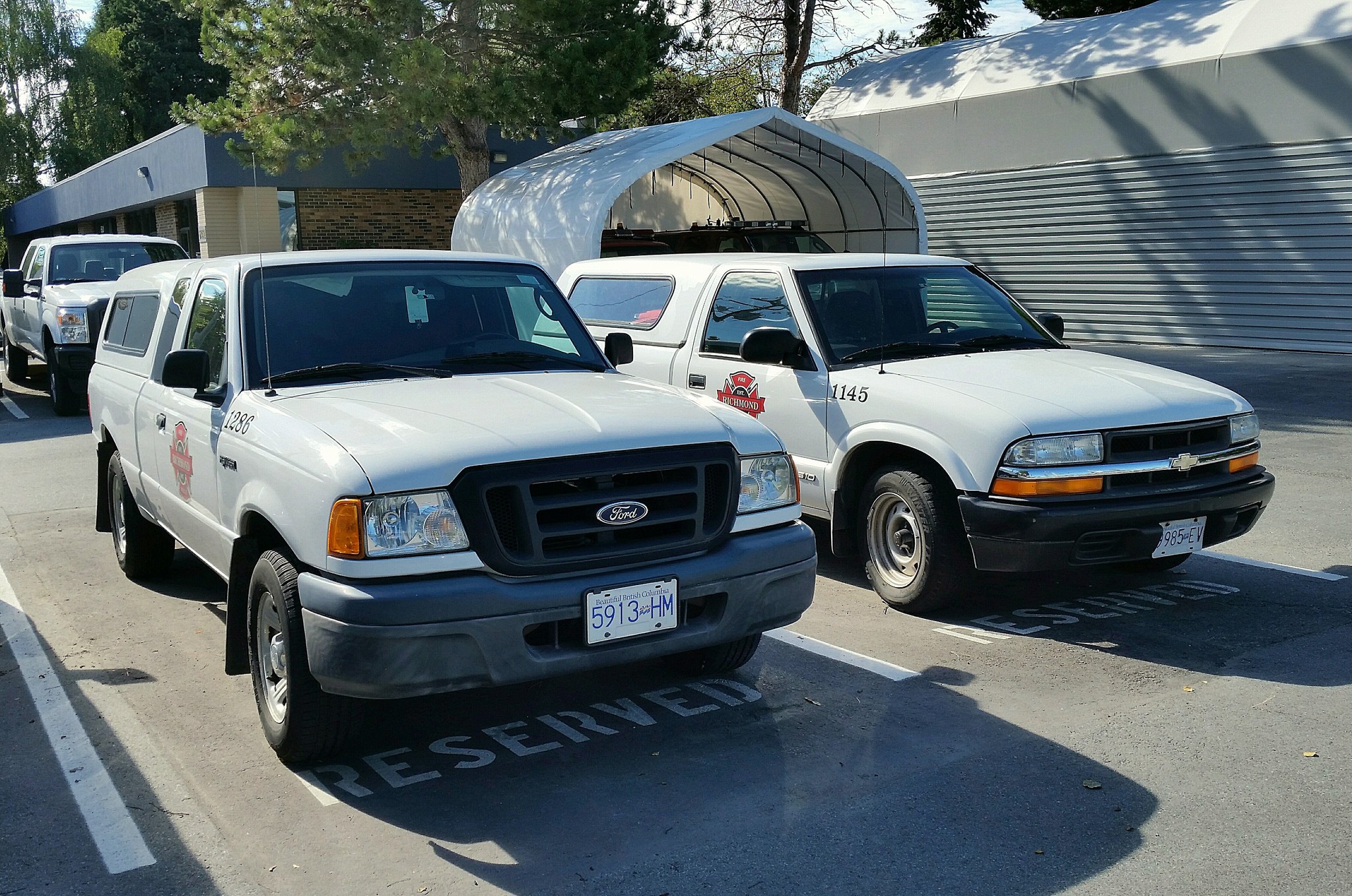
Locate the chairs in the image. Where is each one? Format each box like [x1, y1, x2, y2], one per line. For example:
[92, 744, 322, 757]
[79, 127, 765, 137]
[824, 289, 873, 347]
[54, 254, 118, 279]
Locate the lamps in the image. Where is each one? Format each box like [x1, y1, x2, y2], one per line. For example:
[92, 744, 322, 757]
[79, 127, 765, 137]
[490, 151, 508, 164]
[137, 166, 148, 178]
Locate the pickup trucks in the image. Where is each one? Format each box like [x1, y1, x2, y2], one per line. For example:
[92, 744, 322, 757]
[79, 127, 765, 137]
[0, 234, 191, 416]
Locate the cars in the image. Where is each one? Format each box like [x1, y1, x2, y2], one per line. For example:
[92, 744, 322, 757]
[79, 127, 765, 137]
[0, 267, 4, 280]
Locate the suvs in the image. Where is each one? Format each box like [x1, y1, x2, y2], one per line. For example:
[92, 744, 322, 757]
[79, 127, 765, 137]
[85, 248, 818, 763]
[528, 250, 1275, 607]
